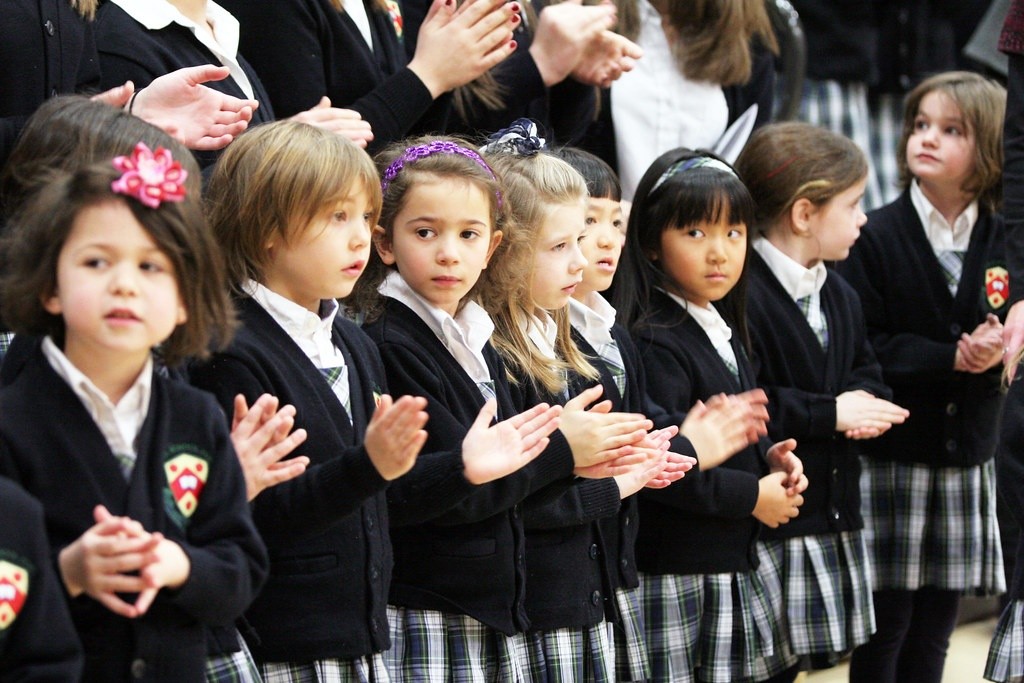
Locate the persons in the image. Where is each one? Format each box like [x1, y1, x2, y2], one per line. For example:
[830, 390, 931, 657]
[0, 0, 1024, 683]
[611, 146, 810, 683]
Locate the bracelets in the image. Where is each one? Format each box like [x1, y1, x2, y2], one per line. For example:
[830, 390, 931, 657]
[128, 87, 140, 117]
